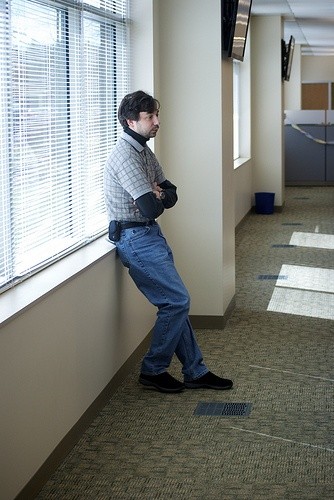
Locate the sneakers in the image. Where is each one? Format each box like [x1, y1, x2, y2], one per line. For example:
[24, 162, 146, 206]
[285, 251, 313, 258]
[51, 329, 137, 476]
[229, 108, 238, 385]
[138, 371, 185, 392]
[185, 371, 233, 390]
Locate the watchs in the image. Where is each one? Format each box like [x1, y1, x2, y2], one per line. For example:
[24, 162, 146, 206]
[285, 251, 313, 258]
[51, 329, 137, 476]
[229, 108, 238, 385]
[159, 191, 165, 200]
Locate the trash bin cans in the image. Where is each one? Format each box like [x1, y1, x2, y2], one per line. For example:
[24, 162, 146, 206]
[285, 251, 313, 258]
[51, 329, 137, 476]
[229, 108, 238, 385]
[255, 192, 275, 214]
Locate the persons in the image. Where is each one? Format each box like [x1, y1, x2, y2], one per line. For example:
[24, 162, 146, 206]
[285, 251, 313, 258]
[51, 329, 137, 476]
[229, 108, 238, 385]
[103, 90, 234, 394]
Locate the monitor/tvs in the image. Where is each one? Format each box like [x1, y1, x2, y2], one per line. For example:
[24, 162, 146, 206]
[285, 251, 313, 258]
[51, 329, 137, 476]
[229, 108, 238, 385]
[284, 35, 295, 81]
[227, 0, 252, 61]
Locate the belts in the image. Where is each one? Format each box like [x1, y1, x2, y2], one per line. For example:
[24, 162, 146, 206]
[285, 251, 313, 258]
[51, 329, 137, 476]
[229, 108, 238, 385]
[120, 222, 147, 229]
